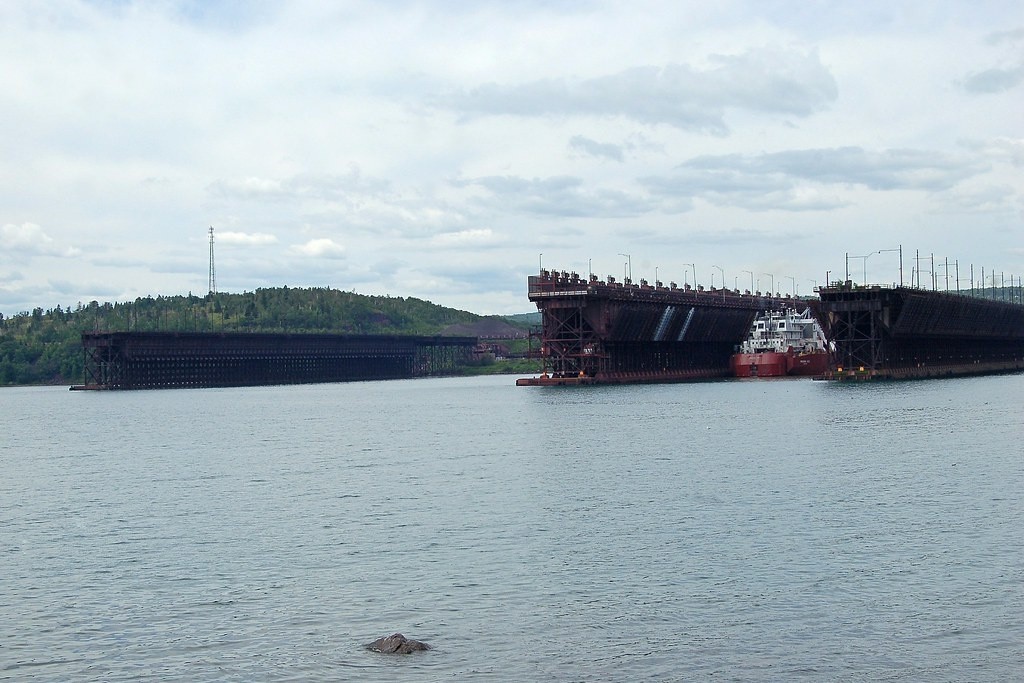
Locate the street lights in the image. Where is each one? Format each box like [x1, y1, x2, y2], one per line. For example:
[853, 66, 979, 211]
[712, 265, 726, 301]
[625, 263, 628, 285]
[784, 276, 794, 298]
[762, 273, 773, 295]
[742, 271, 753, 296]
[539, 254, 542, 278]
[734, 277, 737, 293]
[618, 253, 631, 285]
[684, 270, 688, 294]
[825, 245, 1024, 303]
[655, 266, 659, 289]
[588, 258, 592, 282]
[682, 263, 696, 296]
[809, 278, 817, 296]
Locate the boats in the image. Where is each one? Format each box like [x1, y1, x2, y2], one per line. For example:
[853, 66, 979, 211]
[731, 307, 834, 378]
